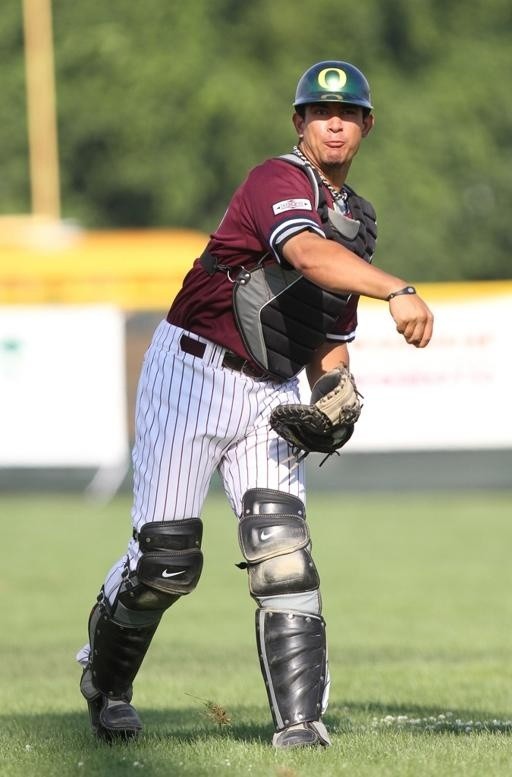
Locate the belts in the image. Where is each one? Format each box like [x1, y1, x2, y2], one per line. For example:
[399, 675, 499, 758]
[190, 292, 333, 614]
[180, 335, 285, 384]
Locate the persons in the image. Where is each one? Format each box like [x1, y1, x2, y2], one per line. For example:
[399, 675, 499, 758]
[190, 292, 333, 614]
[72, 60, 435, 752]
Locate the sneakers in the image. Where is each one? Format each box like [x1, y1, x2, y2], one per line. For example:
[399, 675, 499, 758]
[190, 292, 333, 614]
[87, 697, 138, 742]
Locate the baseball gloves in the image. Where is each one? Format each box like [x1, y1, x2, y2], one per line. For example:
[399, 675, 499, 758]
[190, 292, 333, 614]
[270, 366, 363, 468]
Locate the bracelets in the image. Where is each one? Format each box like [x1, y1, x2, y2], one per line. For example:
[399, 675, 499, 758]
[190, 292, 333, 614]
[386, 285, 417, 302]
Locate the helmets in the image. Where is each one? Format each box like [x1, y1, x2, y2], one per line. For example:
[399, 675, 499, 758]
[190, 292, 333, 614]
[293, 61, 373, 114]
